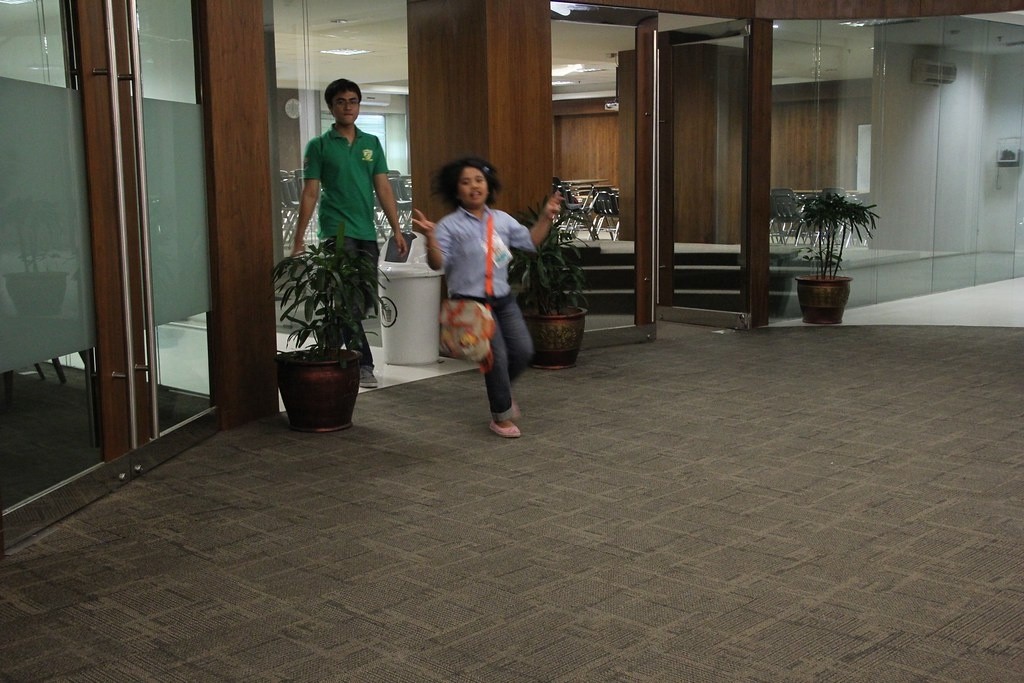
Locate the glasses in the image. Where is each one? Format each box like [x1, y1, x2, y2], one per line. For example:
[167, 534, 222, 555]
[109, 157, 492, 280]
[335, 98, 359, 105]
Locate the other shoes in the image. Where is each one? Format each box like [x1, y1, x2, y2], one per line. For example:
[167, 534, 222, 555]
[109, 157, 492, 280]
[358, 367, 379, 388]
[489, 420, 521, 438]
[507, 398, 522, 418]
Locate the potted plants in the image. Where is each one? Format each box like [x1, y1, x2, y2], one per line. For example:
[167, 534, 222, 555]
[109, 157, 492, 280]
[507, 195, 587, 369]
[271, 221, 394, 436]
[794, 193, 878, 323]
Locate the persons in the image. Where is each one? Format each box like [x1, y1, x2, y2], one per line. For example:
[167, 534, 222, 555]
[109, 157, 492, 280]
[291, 78, 408, 388]
[412, 157, 563, 440]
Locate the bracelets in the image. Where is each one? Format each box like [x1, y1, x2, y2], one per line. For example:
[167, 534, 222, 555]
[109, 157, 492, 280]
[427, 245, 441, 253]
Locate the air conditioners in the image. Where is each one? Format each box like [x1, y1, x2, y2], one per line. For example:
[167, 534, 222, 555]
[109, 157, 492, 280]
[913, 58, 957, 84]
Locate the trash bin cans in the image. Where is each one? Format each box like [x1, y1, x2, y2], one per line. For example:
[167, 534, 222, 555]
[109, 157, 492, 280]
[378, 230, 444, 367]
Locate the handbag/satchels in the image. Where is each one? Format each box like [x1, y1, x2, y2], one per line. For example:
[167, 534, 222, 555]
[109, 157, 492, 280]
[437, 299, 496, 372]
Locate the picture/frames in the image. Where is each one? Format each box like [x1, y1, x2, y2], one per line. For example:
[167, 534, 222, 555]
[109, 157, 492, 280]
[997, 137, 1023, 163]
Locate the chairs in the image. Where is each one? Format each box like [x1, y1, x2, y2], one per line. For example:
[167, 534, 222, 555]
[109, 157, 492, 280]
[279, 168, 413, 247]
[550, 176, 621, 242]
[769, 188, 865, 247]
